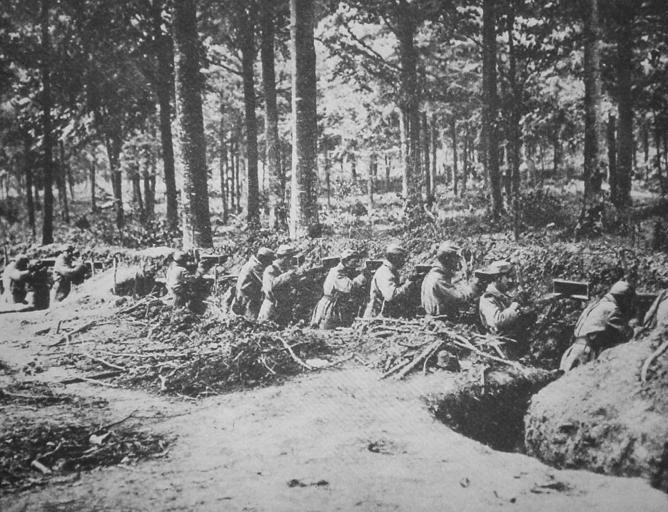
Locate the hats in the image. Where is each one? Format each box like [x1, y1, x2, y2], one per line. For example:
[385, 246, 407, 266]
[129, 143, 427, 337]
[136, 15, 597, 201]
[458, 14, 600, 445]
[62, 245, 76, 252]
[472, 258, 518, 280]
[435, 239, 464, 259]
[12, 253, 44, 271]
[256, 245, 276, 261]
[609, 278, 636, 300]
[275, 243, 295, 258]
[385, 243, 411, 256]
[340, 248, 361, 262]
[173, 248, 189, 263]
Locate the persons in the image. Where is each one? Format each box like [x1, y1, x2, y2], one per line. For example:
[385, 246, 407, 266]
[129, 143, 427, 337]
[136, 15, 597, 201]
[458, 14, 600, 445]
[1, 255, 46, 302]
[558, 281, 636, 374]
[474, 260, 534, 359]
[165, 251, 208, 310]
[364, 245, 414, 321]
[256, 246, 308, 323]
[312, 249, 371, 329]
[49, 245, 88, 304]
[420, 240, 478, 323]
[233, 247, 275, 323]
